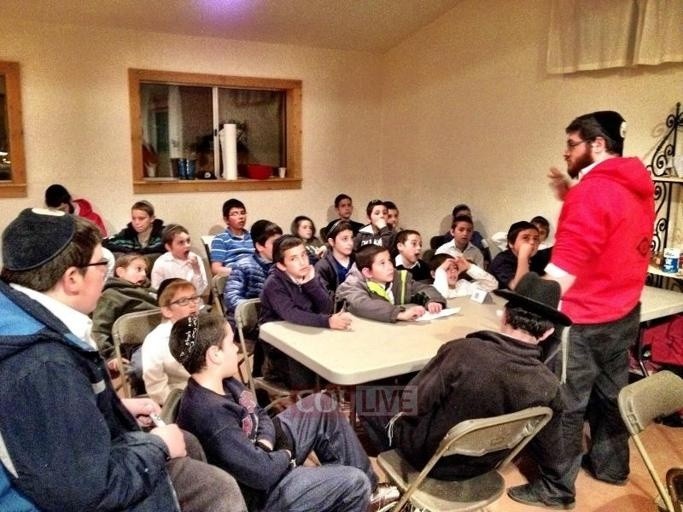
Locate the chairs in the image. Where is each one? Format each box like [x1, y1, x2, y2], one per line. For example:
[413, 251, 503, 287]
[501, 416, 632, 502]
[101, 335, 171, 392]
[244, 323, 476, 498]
[111, 236, 317, 461]
[616, 370, 683, 512]
[376, 407, 552, 512]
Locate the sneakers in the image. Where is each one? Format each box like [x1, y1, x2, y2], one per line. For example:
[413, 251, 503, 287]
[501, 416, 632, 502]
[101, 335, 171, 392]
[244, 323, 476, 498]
[508, 483, 576, 509]
[369, 482, 402, 510]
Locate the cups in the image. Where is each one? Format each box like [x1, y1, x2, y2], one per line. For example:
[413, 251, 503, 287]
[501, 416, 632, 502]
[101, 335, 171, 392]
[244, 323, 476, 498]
[277, 167, 287, 179]
[177, 156, 194, 178]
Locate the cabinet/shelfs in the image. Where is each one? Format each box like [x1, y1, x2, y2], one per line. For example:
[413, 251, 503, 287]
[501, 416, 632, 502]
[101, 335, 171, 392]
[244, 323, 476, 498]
[645, 102, 682, 292]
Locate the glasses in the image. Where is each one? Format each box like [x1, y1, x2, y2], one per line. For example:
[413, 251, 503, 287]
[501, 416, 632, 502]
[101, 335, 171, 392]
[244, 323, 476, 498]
[82, 258, 109, 268]
[163, 296, 201, 307]
[568, 140, 585, 149]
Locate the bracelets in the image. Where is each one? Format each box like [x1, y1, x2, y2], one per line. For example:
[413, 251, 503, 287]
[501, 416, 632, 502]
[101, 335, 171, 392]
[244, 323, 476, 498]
[252, 440, 273, 454]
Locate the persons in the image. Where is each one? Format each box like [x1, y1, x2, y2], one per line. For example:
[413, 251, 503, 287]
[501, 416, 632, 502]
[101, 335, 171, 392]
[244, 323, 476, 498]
[140, 127, 157, 177]
[91, 192, 550, 428]
[507, 111, 655, 509]
[169, 312, 404, 512]
[360, 271, 566, 481]
[45, 185, 107, 241]
[0, 207, 248, 512]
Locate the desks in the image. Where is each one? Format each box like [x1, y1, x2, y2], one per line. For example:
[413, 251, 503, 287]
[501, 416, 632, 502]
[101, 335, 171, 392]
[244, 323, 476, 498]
[258, 291, 509, 386]
[634, 284, 683, 363]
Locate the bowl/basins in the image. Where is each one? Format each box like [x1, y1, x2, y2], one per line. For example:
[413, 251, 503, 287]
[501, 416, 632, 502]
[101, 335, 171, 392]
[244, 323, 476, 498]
[247, 164, 272, 179]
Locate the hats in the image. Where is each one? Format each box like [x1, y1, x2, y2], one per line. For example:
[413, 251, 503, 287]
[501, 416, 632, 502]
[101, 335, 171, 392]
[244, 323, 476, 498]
[1, 207, 75, 271]
[493, 272, 572, 327]
[593, 111, 625, 142]
[170, 315, 200, 364]
[157, 278, 180, 297]
[166, 224, 177, 232]
